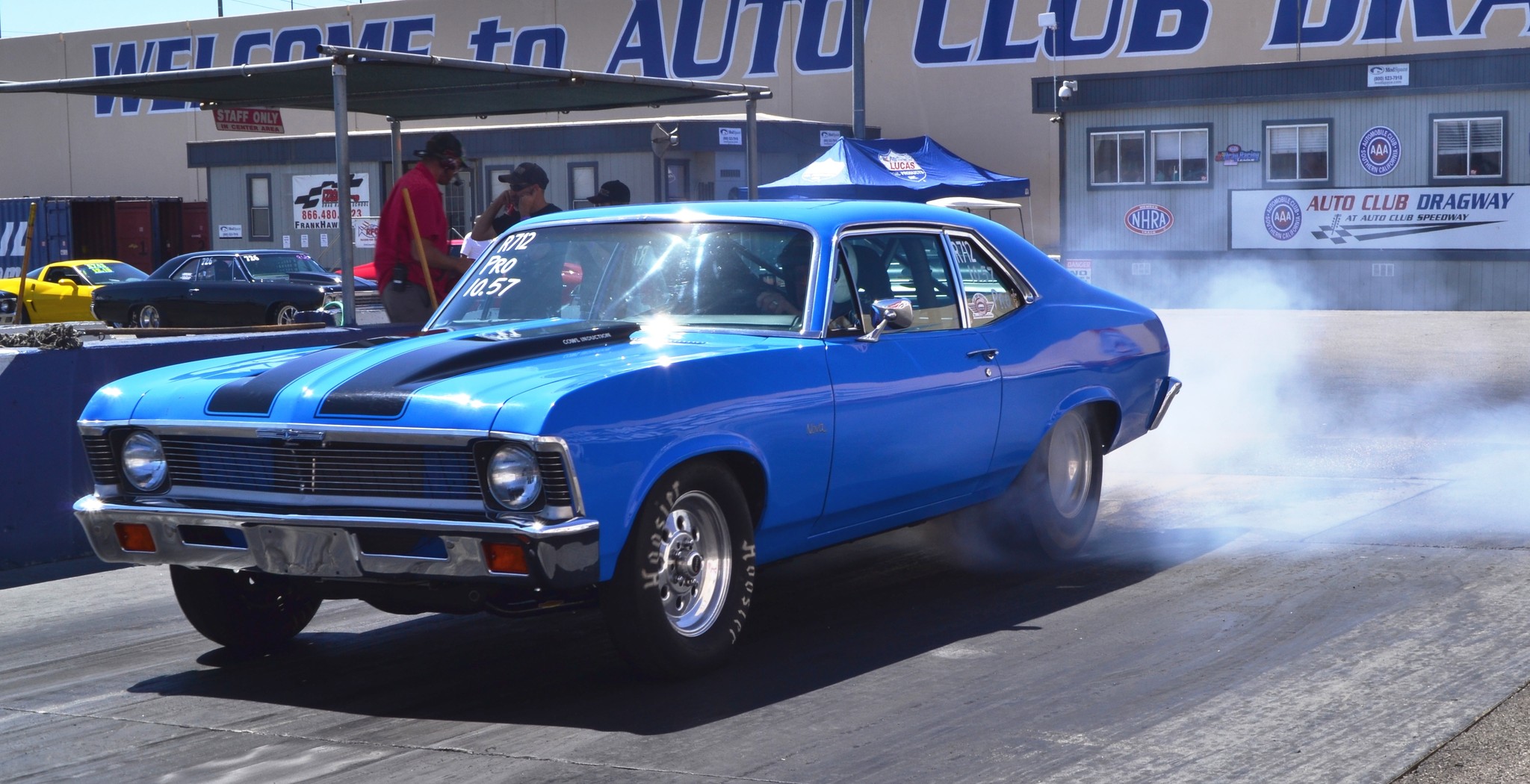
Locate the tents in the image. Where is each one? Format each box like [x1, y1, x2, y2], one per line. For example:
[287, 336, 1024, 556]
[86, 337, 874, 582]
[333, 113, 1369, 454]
[737, 136, 1034, 250]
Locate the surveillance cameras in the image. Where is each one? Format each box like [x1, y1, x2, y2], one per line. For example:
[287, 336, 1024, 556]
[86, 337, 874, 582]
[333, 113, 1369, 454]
[1058, 86, 1071, 102]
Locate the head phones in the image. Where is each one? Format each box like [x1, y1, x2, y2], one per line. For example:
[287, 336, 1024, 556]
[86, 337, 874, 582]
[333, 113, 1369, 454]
[437, 131, 458, 169]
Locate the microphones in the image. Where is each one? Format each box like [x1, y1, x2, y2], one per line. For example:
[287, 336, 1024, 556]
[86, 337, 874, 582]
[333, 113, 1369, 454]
[447, 164, 463, 186]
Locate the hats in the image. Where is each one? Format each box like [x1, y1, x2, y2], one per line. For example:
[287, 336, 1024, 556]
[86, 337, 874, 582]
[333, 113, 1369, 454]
[425, 132, 468, 170]
[587, 180, 631, 202]
[498, 163, 549, 188]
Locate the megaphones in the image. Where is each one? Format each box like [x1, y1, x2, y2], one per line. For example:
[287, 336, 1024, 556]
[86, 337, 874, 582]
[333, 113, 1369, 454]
[651, 122, 678, 158]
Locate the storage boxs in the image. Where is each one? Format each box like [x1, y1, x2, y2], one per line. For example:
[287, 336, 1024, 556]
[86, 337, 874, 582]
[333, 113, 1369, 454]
[0, 195, 211, 282]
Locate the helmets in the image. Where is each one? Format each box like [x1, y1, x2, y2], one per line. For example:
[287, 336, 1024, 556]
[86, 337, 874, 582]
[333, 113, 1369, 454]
[781, 236, 857, 303]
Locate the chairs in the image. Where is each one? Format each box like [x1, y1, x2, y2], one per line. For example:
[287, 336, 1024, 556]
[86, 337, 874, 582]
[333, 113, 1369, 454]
[852, 245, 897, 317]
[214, 260, 232, 281]
[50, 270, 66, 283]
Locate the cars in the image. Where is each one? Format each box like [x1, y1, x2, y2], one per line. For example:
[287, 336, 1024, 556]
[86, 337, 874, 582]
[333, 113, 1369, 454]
[0, 259, 150, 327]
[76, 197, 1182, 674]
[88, 250, 383, 329]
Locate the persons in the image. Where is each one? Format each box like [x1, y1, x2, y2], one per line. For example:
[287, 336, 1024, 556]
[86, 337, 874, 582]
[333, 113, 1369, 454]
[579, 179, 631, 318]
[460, 215, 493, 259]
[756, 234, 877, 331]
[374, 133, 476, 323]
[472, 163, 569, 319]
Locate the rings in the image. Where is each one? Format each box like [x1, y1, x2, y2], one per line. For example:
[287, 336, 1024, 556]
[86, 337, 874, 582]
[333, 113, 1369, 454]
[772, 301, 779, 306]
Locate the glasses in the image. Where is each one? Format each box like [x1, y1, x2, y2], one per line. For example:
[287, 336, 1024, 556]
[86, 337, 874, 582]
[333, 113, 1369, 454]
[509, 183, 533, 191]
